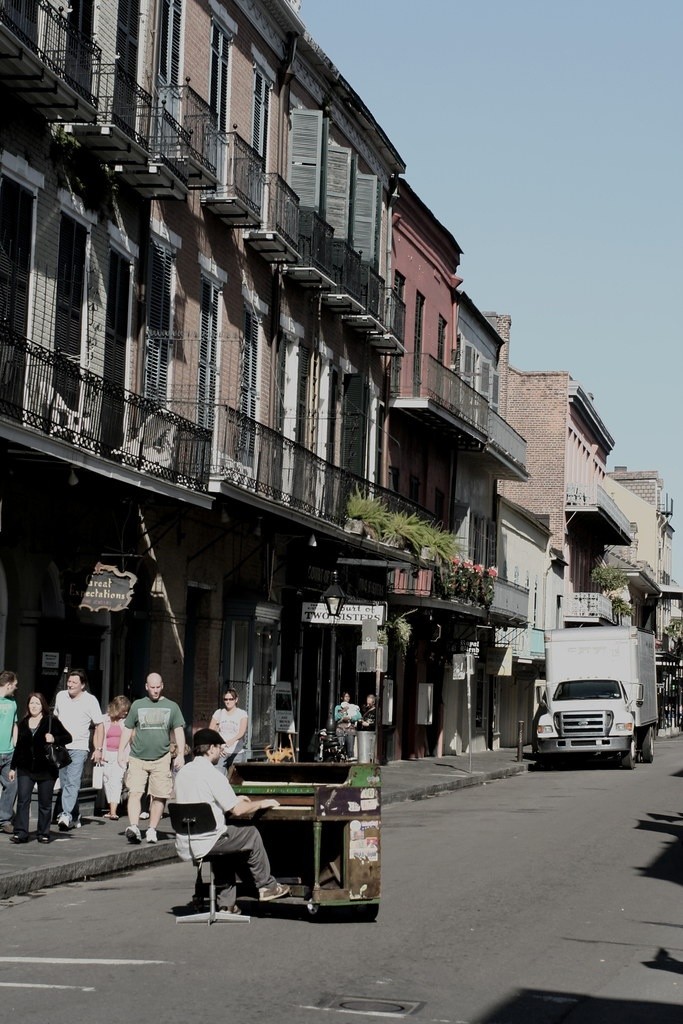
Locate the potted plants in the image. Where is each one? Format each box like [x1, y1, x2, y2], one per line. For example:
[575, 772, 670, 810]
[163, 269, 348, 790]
[375, 608, 419, 657]
[345, 481, 468, 572]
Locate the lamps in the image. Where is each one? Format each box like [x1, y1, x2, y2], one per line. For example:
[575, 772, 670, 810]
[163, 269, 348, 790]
[221, 505, 230, 523]
[254, 516, 263, 537]
[308, 532, 317, 547]
[67, 467, 79, 486]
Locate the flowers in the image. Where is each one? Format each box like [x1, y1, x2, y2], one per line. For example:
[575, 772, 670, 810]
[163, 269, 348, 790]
[430, 554, 498, 610]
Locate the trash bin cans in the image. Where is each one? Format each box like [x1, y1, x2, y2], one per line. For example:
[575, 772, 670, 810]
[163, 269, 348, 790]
[356, 731, 377, 763]
[367, 723, 396, 765]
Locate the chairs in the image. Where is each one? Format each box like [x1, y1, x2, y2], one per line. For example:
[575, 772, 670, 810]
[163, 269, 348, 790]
[167, 802, 251, 925]
[116, 409, 178, 476]
[40, 381, 90, 446]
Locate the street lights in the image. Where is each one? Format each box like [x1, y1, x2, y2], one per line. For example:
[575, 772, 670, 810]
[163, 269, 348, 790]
[322, 570, 348, 763]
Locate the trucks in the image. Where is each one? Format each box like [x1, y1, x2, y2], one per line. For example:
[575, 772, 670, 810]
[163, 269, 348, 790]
[536, 626, 659, 771]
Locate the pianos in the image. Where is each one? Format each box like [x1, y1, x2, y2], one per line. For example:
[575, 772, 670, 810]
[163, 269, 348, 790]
[226, 760, 384, 915]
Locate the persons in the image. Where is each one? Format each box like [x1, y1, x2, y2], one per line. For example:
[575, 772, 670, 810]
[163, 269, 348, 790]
[358, 694, 378, 732]
[173, 728, 290, 914]
[90, 695, 134, 822]
[8, 692, 73, 844]
[117, 672, 186, 844]
[0, 670, 19, 833]
[333, 691, 361, 759]
[51, 670, 105, 829]
[206, 688, 248, 767]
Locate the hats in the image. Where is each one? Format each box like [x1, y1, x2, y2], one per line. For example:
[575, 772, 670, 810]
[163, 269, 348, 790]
[193, 729, 226, 744]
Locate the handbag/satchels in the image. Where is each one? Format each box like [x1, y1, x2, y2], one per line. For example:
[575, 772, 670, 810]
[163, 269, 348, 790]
[45, 742, 72, 770]
[93, 762, 103, 789]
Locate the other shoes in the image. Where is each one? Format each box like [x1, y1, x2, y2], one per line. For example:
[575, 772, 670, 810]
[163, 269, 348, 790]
[10, 835, 23, 843]
[139, 812, 150, 819]
[38, 834, 49, 842]
[103, 813, 111, 817]
[110, 814, 119, 819]
[1, 823, 14, 833]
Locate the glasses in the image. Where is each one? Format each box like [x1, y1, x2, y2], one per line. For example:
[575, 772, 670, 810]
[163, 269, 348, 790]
[213, 745, 224, 752]
[223, 698, 235, 701]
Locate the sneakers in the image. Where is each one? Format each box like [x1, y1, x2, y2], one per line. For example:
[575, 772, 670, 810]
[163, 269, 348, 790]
[57, 812, 72, 831]
[68, 819, 81, 828]
[259, 883, 291, 901]
[146, 828, 158, 843]
[219, 905, 241, 915]
[125, 825, 141, 844]
